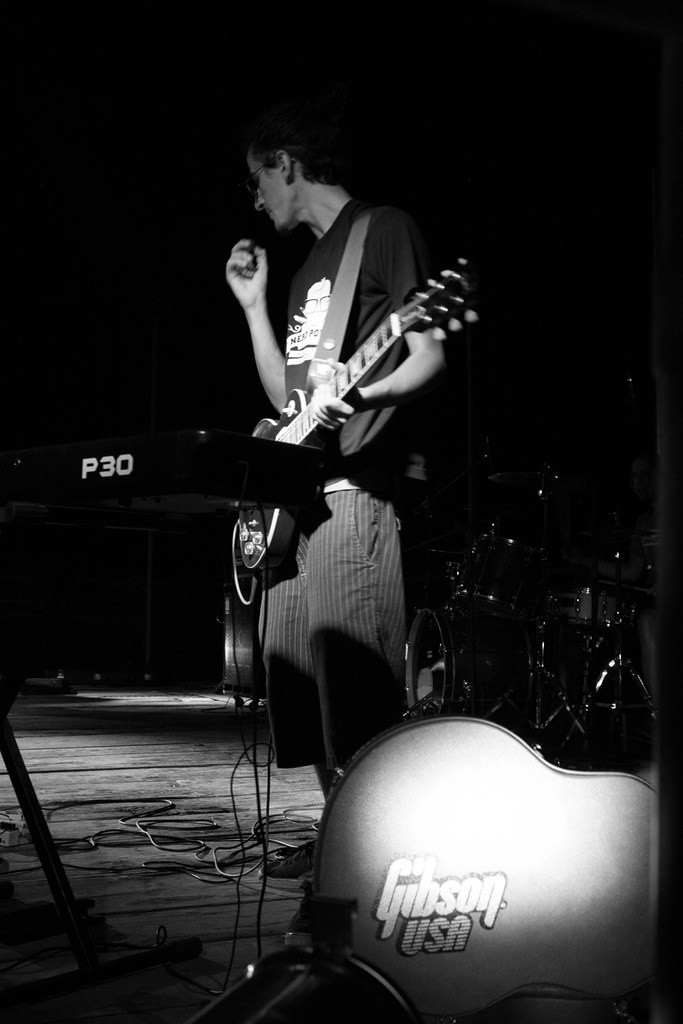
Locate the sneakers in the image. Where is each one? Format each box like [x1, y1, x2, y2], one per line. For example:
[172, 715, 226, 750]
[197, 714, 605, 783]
[258, 841, 315, 888]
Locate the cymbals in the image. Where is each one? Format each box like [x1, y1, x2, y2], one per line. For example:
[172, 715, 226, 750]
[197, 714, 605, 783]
[577, 525, 655, 538]
[487, 467, 568, 488]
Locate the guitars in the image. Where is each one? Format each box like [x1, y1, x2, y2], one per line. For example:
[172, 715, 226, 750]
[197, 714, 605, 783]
[236, 256, 482, 571]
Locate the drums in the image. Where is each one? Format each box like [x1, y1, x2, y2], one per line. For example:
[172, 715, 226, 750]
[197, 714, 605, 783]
[550, 583, 642, 627]
[453, 532, 550, 622]
[404, 607, 535, 733]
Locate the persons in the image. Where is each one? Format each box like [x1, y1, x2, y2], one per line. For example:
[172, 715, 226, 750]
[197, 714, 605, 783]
[223, 113, 446, 891]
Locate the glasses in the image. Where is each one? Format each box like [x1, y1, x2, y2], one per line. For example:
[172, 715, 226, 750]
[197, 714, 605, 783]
[244, 156, 295, 197]
